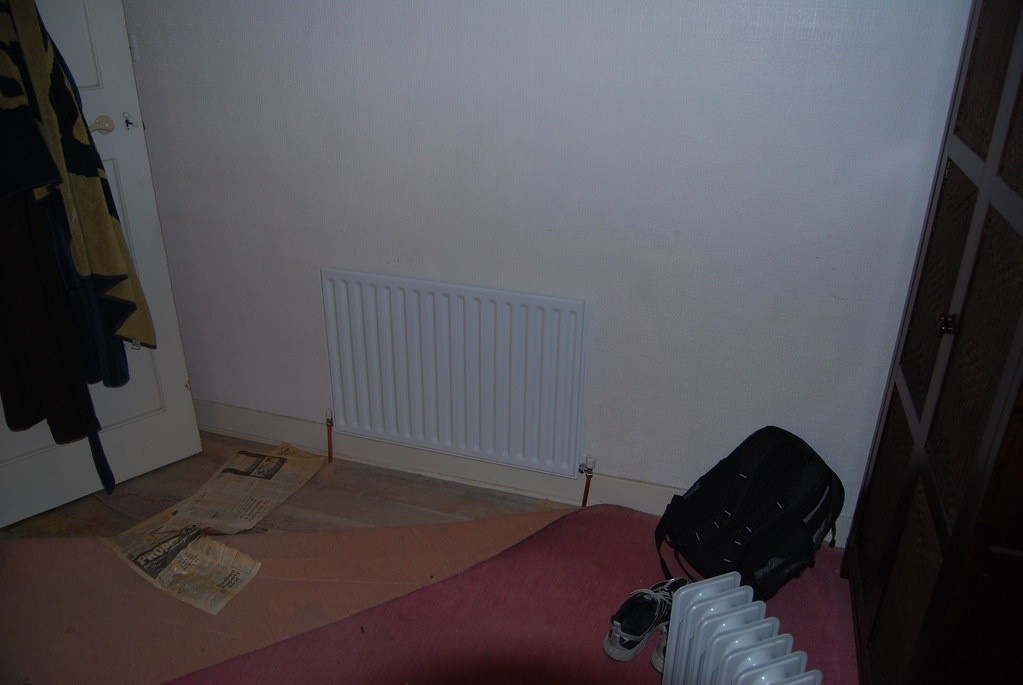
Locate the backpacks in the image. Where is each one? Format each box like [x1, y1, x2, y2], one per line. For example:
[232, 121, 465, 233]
[656, 425, 845, 592]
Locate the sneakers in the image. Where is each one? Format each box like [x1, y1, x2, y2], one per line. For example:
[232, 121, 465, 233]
[602, 577, 692, 674]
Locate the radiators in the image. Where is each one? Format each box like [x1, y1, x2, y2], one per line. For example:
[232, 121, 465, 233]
[322, 266, 587, 480]
[662, 572, 823, 685]
